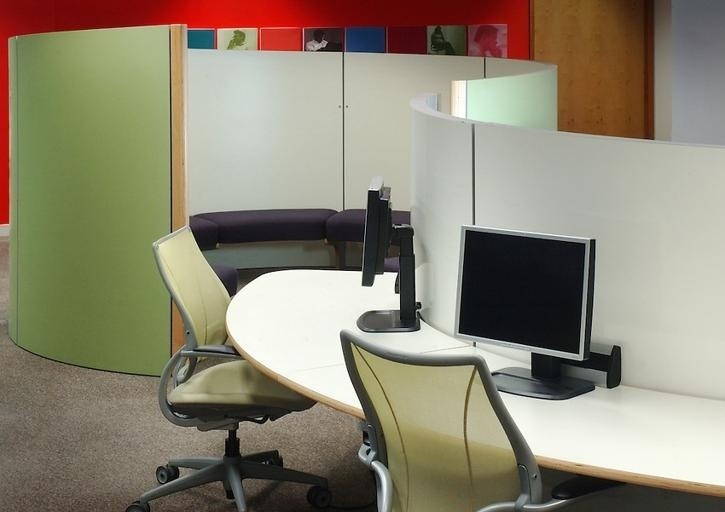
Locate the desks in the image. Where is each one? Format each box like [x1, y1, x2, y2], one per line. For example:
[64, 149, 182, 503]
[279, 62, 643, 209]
[224, 267, 725, 499]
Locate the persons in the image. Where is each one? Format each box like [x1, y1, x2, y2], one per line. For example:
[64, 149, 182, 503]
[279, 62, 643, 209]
[430, 30, 456, 55]
[469, 26, 503, 57]
[224, 30, 250, 50]
[305, 29, 330, 51]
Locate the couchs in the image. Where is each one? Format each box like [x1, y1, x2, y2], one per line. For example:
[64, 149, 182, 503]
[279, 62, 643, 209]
[189, 208, 423, 296]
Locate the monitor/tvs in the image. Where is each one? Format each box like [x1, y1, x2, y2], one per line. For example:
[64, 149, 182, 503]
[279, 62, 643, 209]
[357, 177, 421, 333]
[454, 225, 596, 400]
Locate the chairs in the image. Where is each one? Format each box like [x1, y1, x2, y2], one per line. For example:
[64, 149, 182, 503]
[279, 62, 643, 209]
[124, 222, 335, 511]
[338, 329, 628, 512]
